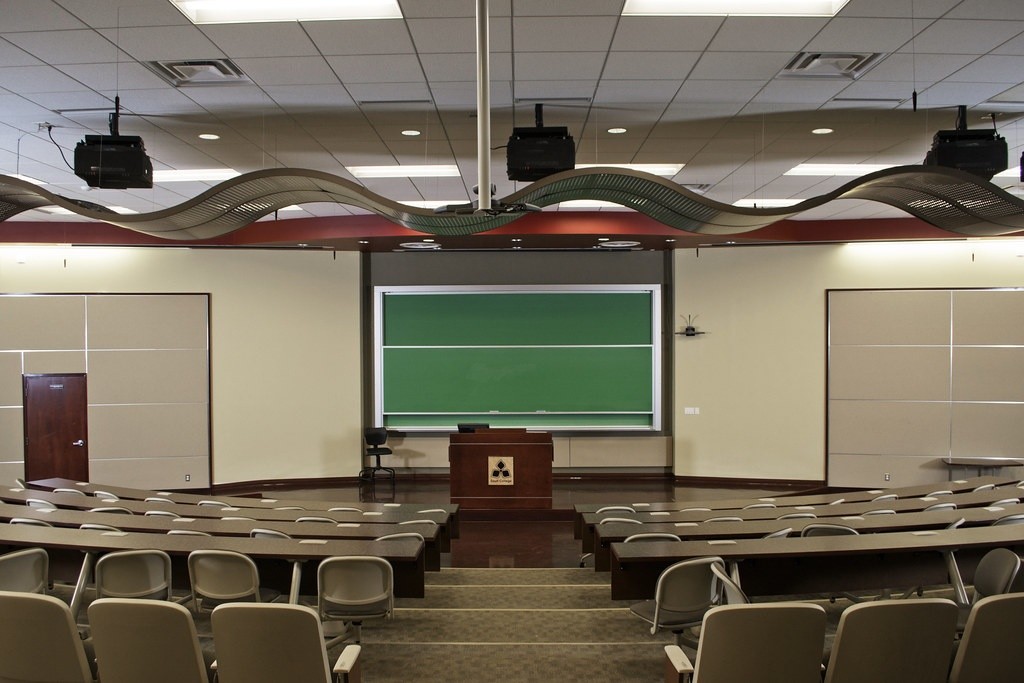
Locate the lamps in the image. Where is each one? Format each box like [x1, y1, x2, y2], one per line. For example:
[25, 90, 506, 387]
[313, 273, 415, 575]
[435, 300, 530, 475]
[505, 103, 576, 182]
[922, 104, 1009, 181]
[73, 114, 153, 188]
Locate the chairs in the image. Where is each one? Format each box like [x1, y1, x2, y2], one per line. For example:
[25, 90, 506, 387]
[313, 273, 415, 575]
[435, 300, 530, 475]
[596, 480, 1024, 683]
[0, 476, 446, 683]
[359, 427, 396, 478]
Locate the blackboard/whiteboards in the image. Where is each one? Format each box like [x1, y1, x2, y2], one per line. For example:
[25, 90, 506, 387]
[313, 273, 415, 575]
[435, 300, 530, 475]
[367, 283, 663, 433]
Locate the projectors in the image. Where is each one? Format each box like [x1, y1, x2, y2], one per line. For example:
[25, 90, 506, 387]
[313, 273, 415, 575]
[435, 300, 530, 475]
[74, 147, 153, 190]
[506, 136, 575, 182]
[924, 137, 1008, 176]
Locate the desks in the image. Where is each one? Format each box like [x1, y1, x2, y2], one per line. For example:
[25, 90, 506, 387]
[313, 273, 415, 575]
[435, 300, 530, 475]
[0, 501, 441, 573]
[609, 522, 1024, 599]
[0, 485, 452, 554]
[573, 476, 1021, 540]
[0, 522, 426, 598]
[580, 483, 1024, 568]
[943, 458, 1024, 482]
[598, 504, 1024, 573]
[28, 476, 460, 541]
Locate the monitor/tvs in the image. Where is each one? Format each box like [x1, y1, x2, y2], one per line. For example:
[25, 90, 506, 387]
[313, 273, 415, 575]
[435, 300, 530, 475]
[458, 423, 489, 434]
